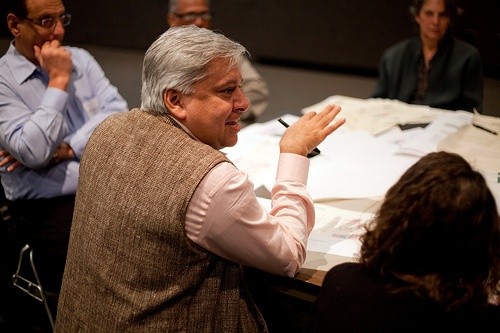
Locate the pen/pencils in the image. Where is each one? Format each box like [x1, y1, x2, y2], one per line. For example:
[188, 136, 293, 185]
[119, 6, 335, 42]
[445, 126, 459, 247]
[473, 123, 496, 134]
[278, 118, 320, 153]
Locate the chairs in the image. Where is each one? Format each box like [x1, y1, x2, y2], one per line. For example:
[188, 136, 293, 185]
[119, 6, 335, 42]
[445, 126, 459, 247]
[0, 180, 57, 331]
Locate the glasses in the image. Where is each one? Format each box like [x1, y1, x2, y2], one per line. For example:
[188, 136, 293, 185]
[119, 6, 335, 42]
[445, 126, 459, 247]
[27, 14, 71, 28]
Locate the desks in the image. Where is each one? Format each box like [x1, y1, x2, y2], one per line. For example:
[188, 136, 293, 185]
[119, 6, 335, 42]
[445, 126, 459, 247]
[220, 93, 500, 307]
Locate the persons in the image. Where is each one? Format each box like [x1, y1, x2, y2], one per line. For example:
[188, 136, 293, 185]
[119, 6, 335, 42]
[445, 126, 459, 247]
[315, 151, 500, 333]
[54, 24, 346, 333]
[165, 0, 270, 130]
[0, 0, 130, 322]
[377, 0, 481, 111]
